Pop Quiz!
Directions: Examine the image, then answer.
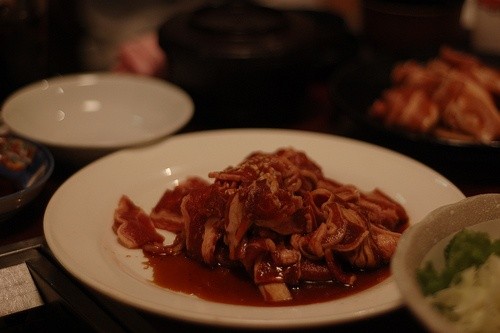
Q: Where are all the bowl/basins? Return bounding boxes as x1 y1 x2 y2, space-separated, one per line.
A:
401 128 500 197
391 191 500 333
1 135 55 221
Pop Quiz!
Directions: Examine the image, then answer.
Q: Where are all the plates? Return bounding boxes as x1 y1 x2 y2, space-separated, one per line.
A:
3 72 195 157
43 128 468 328
